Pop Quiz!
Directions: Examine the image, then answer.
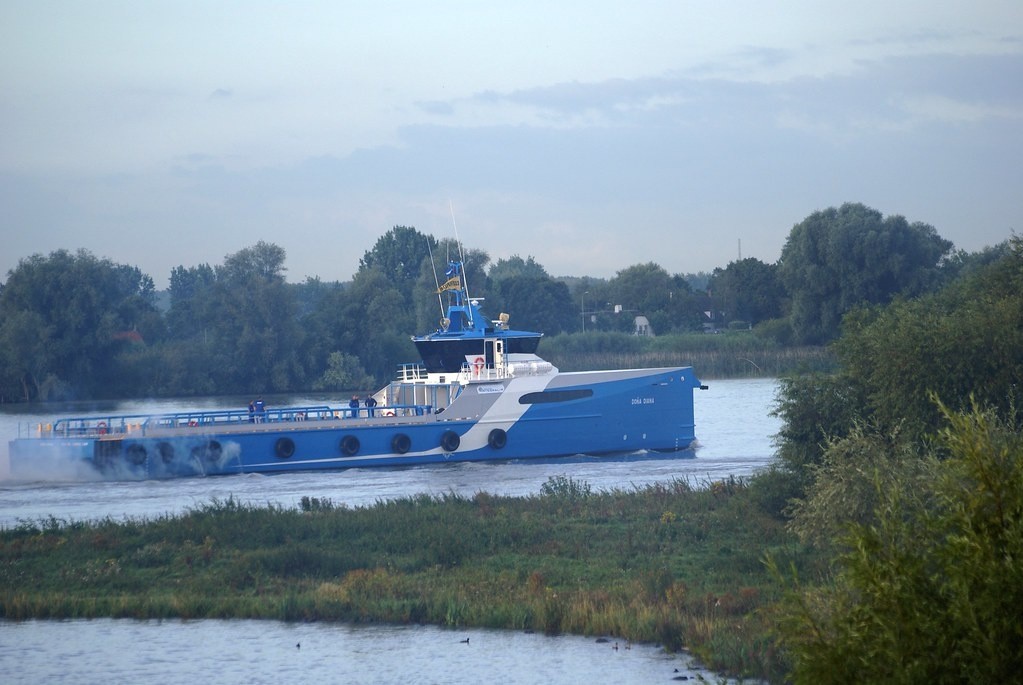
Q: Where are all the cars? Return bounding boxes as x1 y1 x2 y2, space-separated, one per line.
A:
700 327 719 334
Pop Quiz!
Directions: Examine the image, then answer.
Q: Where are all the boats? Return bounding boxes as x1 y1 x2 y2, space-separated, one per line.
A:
10 202 704 489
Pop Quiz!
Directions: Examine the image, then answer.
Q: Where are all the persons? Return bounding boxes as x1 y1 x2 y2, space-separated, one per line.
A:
365 393 377 417
248 396 266 422
349 393 360 417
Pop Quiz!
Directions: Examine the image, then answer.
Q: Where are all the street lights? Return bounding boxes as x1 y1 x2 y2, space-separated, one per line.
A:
582 290 589 332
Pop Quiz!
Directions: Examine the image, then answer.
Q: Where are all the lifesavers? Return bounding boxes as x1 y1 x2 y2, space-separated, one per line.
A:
386 412 394 416
474 357 485 369
154 442 175 463
487 428 507 450
340 434 361 455
440 430 461 452
275 436 295 459
204 441 223 460
125 443 147 465
96 421 107 434
391 433 411 455
188 421 198 427
